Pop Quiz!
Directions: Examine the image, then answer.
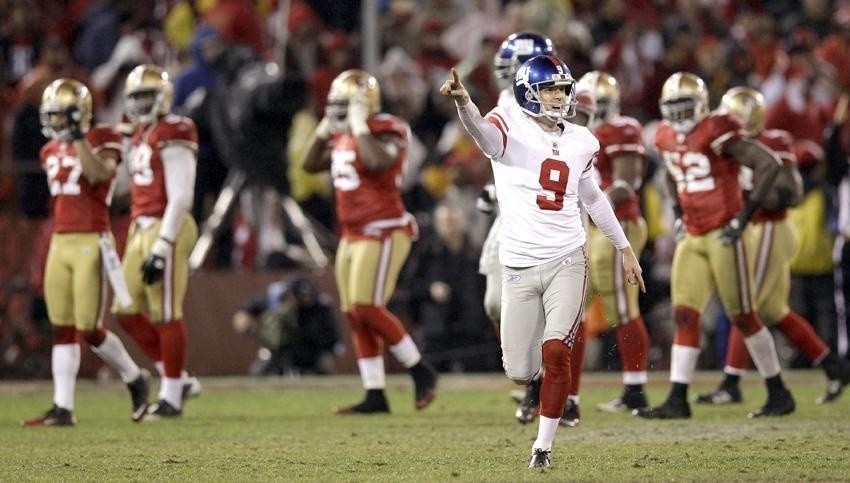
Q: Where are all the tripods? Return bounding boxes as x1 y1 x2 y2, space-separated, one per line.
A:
188 181 338 273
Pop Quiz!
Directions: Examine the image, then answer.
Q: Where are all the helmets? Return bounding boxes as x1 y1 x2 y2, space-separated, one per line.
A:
39 79 93 143
573 70 622 130
494 31 558 80
657 71 710 134
122 64 173 125
324 68 381 136
721 86 768 138
513 55 577 122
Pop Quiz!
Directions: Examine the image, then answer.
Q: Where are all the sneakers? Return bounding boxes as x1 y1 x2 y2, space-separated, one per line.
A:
529 448 552 469
510 373 580 427
632 394 691 419
21 404 78 426
332 388 390 414
815 357 850 405
748 391 794 419
595 390 647 413
696 386 741 406
409 359 438 409
126 369 203 422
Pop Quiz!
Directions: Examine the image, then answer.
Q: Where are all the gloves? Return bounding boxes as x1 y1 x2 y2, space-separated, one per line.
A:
717 214 748 246
139 255 166 285
61 105 86 140
346 95 372 137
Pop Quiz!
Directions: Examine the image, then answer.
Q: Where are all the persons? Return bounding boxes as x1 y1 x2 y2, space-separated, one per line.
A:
0 2 850 467
